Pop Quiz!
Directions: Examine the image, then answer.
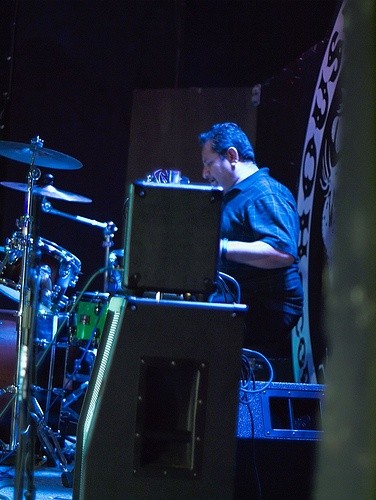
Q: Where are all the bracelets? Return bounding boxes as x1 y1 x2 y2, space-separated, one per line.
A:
221 236 229 261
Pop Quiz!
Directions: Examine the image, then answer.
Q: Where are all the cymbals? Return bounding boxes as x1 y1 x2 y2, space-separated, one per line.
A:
0 139 84 172
0 180 93 204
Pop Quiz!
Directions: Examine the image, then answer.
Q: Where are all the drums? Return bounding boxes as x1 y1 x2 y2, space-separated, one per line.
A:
70 289 110 345
1 311 74 436
1 226 83 316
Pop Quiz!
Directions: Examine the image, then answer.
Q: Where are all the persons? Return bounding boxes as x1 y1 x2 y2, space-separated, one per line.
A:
200 122 303 500
